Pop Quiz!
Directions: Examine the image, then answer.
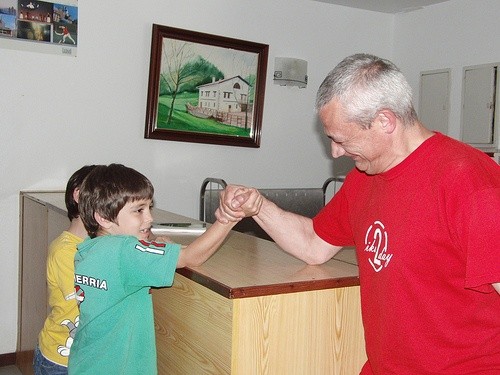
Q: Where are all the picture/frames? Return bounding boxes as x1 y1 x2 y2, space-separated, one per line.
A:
141 21 271 149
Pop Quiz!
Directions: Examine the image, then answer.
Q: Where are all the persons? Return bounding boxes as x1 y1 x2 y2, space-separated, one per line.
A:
215 50 500 375
33 163 103 375
65 162 262 375
0 0 77 46
